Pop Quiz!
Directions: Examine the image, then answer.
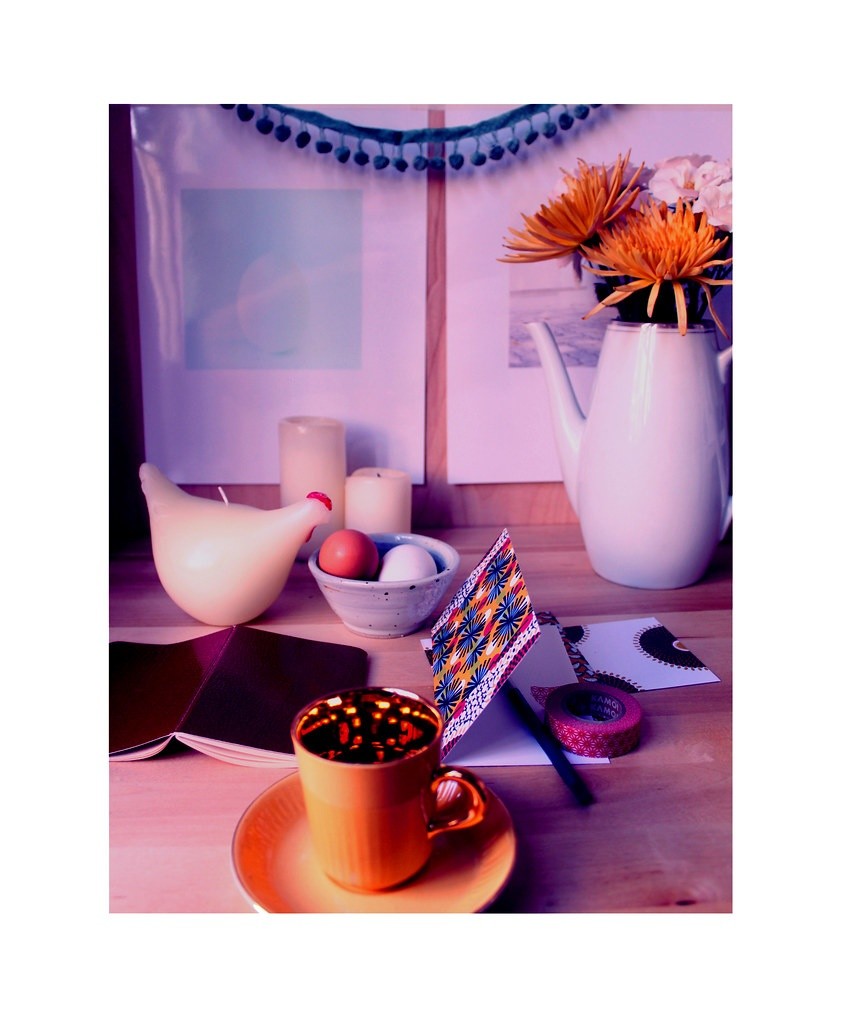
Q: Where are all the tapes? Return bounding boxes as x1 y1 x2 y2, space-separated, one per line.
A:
545 681 645 760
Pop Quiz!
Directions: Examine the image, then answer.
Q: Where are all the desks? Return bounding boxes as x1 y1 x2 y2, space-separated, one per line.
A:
110 521 732 913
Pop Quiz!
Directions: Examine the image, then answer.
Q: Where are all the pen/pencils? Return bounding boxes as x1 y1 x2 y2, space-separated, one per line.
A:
500 681 597 809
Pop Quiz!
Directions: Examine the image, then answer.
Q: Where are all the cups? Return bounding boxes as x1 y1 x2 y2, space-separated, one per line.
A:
292 685 490 894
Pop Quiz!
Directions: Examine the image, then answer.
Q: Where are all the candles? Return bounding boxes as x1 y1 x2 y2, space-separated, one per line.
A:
343 466 412 537
279 414 348 562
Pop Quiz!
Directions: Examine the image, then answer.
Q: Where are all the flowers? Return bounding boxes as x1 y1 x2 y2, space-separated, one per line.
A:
494 149 732 344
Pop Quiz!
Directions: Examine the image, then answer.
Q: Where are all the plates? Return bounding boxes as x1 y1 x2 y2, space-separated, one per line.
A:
231 765 518 913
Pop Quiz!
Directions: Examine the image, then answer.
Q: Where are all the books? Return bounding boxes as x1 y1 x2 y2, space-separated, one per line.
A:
109 623 371 771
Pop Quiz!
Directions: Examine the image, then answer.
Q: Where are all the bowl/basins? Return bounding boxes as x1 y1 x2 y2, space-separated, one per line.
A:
308 531 460 640
